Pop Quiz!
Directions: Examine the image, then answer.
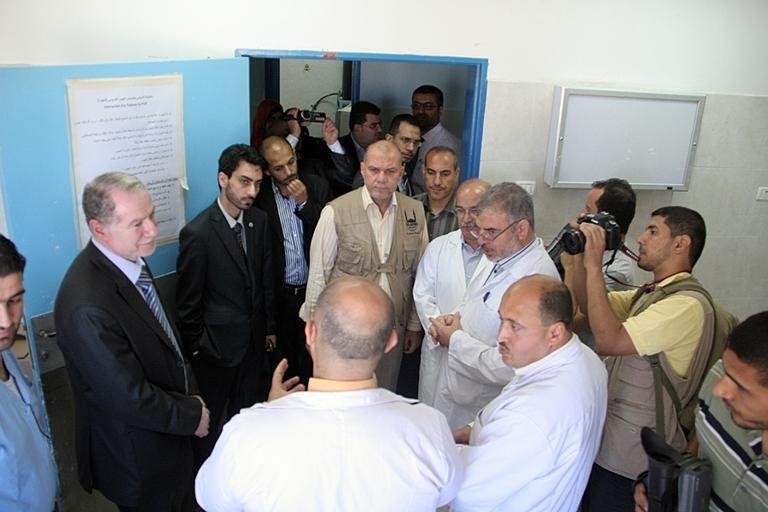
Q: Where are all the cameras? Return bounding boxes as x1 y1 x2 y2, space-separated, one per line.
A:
562 211 622 256
297 109 326 122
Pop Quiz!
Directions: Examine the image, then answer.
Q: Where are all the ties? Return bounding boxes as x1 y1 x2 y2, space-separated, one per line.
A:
229 221 250 264
133 263 185 365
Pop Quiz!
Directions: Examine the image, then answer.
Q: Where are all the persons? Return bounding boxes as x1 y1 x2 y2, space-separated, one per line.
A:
567 207 716 512
52 171 210 511
0 232 63 512
632 309 768 512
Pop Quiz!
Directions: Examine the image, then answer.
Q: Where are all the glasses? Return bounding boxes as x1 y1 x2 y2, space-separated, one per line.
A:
362 120 383 129
410 101 438 112
469 224 503 244
398 135 424 148
